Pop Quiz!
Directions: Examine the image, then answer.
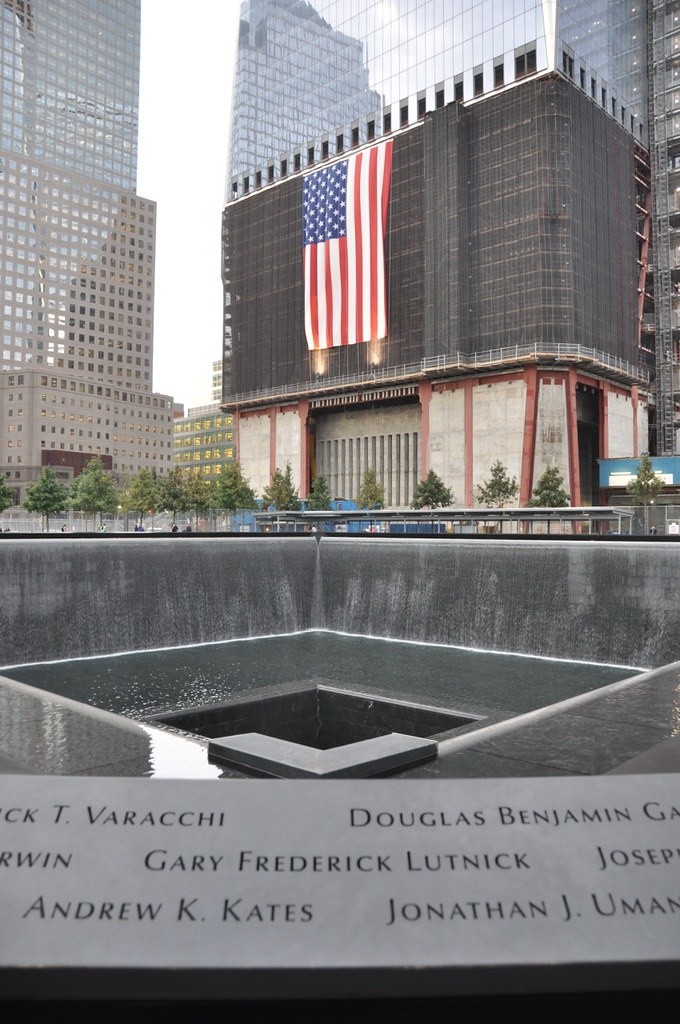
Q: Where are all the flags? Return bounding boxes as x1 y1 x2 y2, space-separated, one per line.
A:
298 138 400 350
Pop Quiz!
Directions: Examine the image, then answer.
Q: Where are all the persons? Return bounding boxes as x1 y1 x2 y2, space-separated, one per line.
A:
649 525 658 537
61 524 66 532
4 525 10 532
309 525 318 533
138 524 144 532
99 524 103 532
605 527 626 537
186 526 192 532
171 524 178 532
279 526 284 531
134 525 138 532
102 523 107 533
